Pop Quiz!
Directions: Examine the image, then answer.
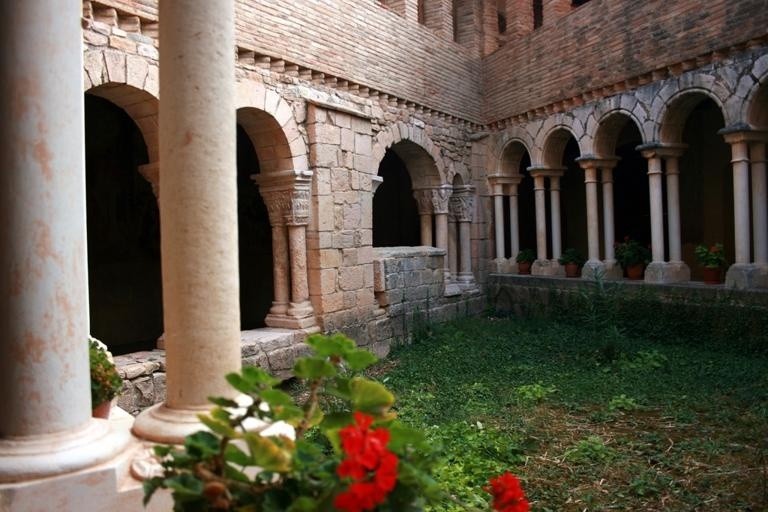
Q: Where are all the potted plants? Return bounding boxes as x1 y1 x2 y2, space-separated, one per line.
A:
614 243 648 281
87 336 126 419
694 243 727 285
515 250 535 273
558 247 583 278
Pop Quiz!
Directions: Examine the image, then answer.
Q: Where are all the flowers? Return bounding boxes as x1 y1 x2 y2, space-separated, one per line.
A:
139 332 534 511
